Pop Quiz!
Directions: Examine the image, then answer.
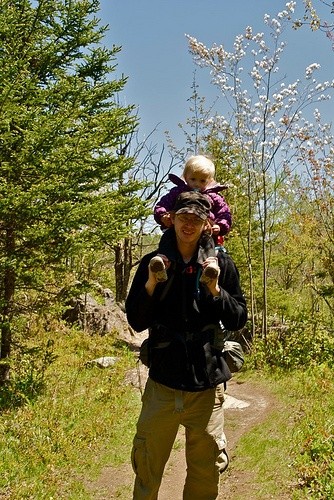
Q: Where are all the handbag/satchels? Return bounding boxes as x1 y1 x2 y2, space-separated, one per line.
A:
222 341 244 373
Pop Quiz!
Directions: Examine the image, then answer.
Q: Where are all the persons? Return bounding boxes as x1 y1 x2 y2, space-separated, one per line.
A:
148 155 232 284
124 190 248 500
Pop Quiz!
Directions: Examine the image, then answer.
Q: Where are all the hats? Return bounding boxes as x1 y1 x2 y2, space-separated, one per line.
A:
174 191 211 221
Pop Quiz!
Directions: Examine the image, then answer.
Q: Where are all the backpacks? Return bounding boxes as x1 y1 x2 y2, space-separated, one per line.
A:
134 338 150 368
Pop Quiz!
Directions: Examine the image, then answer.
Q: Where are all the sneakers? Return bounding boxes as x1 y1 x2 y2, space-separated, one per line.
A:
150 256 167 282
200 256 221 283
